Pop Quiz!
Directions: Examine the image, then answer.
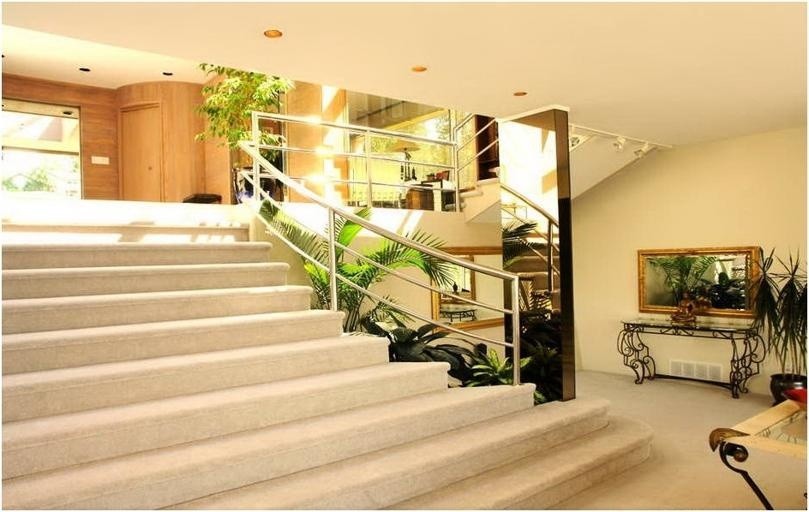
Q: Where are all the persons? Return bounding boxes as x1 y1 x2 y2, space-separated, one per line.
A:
669 290 696 322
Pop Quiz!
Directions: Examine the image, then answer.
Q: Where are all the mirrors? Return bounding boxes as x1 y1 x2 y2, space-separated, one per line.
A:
638 245 760 318
439 257 476 304
431 247 505 335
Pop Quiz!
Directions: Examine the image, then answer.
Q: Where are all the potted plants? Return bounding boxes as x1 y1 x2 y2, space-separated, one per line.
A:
741 246 807 404
647 255 716 322
195 64 294 203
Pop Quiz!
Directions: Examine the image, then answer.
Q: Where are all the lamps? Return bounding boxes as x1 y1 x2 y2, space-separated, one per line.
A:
383 131 421 182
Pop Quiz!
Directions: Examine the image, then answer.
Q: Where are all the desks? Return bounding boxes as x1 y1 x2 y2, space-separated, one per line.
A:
617 318 765 398
440 306 477 322
